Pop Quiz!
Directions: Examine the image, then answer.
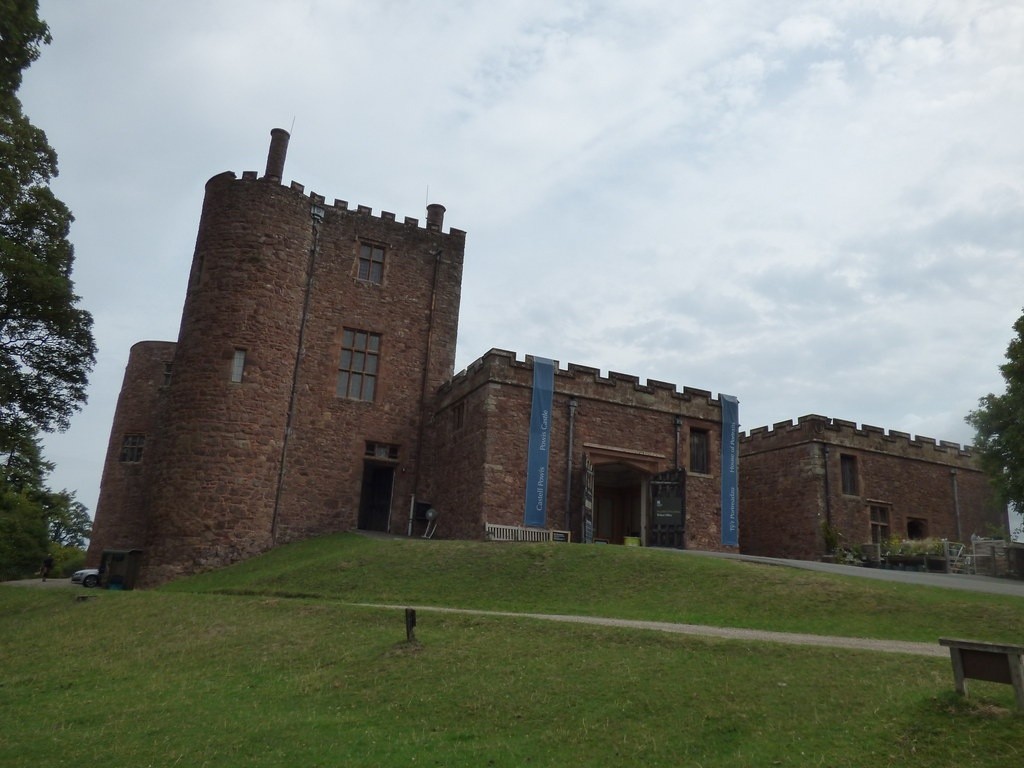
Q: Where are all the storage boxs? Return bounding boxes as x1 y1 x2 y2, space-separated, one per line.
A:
624 536 640 546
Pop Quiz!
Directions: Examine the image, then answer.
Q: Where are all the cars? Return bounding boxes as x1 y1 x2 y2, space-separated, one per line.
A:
71 568 100 589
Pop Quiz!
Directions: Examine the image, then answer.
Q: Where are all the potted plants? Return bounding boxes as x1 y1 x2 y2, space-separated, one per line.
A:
912 540 927 564
885 530 903 569
950 544 959 556
821 521 846 563
901 551 920 571
926 536 947 573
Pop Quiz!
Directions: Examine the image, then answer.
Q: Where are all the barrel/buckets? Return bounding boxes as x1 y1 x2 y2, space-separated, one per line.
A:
624 537 640 546
863 543 880 559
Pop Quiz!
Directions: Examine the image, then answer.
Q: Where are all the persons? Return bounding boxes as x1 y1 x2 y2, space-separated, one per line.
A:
40 553 53 582
